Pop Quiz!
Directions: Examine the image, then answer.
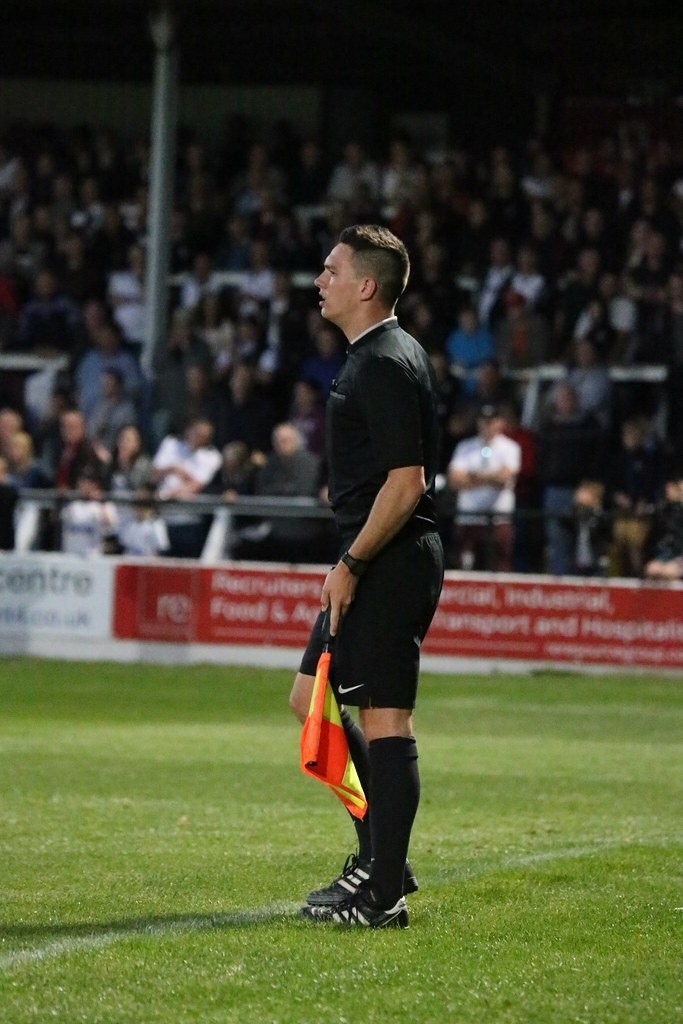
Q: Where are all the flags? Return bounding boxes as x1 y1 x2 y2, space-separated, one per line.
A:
301 652 368 820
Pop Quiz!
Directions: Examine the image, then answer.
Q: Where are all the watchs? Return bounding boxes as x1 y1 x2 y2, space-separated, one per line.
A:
341 552 367 577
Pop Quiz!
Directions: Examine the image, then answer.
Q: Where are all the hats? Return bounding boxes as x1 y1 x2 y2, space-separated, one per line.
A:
478 399 498 421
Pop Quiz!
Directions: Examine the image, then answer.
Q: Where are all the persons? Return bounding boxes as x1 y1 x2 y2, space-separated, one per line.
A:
288 225 443 932
0 94 683 577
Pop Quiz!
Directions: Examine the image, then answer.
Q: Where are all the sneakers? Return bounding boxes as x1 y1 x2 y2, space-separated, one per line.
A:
306 848 418 906
300 886 410 933
